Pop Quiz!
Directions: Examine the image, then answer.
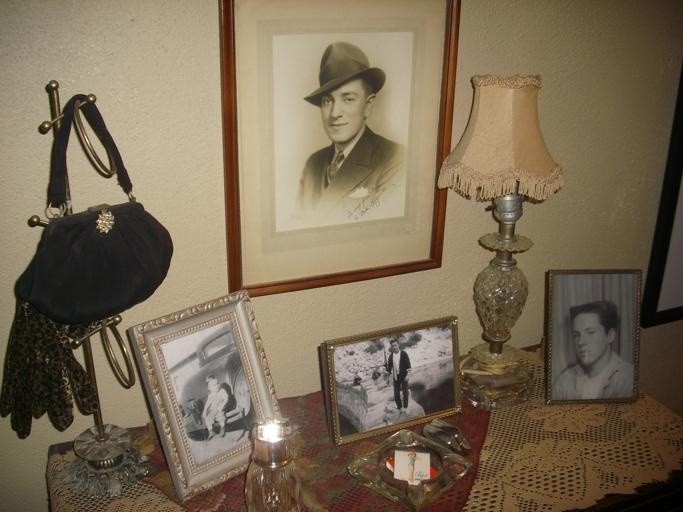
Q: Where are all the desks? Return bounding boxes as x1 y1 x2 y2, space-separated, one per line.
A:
46 344 683 511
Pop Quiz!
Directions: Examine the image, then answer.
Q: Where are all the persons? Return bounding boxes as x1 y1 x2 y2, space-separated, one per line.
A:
551 300 633 398
381 339 412 415
295 39 405 211
201 375 230 440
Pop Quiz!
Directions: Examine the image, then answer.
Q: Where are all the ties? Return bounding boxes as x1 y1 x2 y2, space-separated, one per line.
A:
325 150 344 186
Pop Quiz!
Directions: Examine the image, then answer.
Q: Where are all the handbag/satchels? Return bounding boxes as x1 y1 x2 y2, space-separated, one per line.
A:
14 94 174 324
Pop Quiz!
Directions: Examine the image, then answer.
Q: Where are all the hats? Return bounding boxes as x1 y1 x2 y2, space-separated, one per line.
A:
303 41 386 107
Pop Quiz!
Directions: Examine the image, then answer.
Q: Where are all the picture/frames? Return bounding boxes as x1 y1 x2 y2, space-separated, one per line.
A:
319 316 462 444
546 269 641 403
131 289 283 502
220 1 460 297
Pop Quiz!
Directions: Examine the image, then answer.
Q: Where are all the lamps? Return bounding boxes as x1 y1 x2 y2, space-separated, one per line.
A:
245 418 307 512
437 73 566 412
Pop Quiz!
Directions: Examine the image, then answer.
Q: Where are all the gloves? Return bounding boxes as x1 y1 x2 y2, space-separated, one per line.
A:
0 298 99 440
